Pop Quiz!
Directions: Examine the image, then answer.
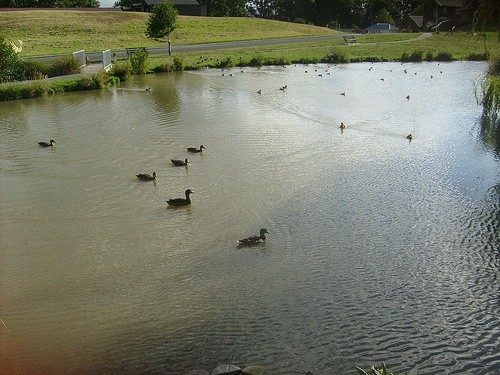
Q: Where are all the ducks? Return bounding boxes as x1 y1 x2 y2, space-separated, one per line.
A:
407 134 412 139
406 95 410 99
340 122 346 128
38 139 56 147
165 189 194 206
257 85 290 96
135 172 157 181
219 67 245 77
186 144 206 153
171 158 188 166
145 88 151 90
237 228 271 244
340 92 346 96
282 63 443 81
